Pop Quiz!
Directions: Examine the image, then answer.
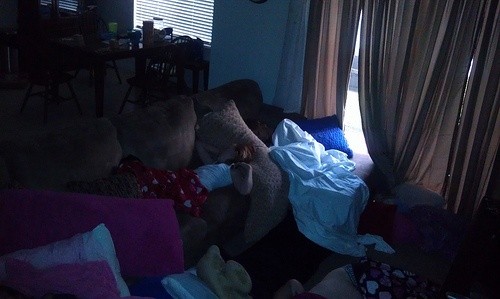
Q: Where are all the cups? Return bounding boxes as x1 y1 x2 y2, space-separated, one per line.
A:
143 21 153 36
108 22 117 33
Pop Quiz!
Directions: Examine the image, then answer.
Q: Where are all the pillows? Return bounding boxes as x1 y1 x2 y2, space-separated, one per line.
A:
294 115 354 158
195 101 286 189
361 184 483 262
0 187 220 299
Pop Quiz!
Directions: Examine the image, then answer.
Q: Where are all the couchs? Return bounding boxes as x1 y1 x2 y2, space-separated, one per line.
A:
0 77 306 268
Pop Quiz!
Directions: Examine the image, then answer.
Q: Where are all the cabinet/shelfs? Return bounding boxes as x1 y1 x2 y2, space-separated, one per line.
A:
16 0 97 74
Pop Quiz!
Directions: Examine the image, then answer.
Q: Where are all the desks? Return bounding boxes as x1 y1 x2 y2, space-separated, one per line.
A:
48 33 184 118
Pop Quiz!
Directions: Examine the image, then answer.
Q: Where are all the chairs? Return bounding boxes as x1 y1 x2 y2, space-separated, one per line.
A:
19 34 83 123
72 11 123 85
117 35 210 115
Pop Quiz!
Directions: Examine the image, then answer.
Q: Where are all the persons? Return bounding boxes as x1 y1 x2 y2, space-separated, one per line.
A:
111 140 258 217
